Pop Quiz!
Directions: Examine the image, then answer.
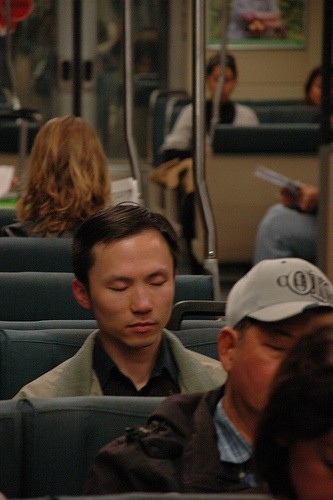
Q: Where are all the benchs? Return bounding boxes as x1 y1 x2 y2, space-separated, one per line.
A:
0 208 230 500
151 89 320 263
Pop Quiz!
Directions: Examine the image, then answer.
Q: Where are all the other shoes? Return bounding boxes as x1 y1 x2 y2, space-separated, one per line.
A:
189 256 210 276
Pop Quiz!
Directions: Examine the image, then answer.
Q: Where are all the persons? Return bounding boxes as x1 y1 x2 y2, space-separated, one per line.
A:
12 199 236 405
0 52 320 277
229 0 285 39
96 258 333 499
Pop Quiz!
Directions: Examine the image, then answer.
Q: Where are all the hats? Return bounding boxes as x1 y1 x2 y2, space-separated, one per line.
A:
225 257 333 329
